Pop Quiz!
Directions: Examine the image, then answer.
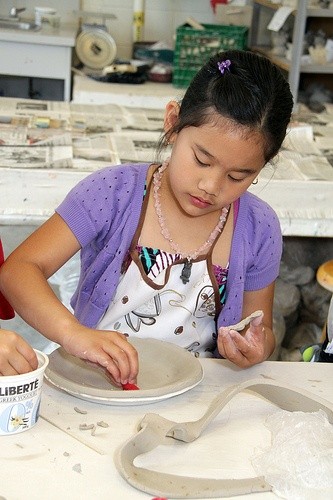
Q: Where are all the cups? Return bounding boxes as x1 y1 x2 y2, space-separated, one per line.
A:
0 347 49 436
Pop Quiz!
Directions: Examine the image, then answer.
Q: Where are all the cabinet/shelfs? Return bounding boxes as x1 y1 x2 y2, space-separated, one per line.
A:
249 0 333 116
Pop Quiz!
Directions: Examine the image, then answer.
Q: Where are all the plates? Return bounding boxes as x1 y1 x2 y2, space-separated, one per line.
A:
144 64 173 83
44 337 204 407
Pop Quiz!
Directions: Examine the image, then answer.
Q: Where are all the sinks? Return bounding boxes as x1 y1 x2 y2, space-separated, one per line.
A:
0 20 40 31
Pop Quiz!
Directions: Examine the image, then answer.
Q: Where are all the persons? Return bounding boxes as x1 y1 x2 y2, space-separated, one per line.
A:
0 238 38 375
0 48 294 385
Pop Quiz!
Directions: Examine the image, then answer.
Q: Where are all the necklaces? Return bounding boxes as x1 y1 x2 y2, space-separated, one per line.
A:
152 157 229 262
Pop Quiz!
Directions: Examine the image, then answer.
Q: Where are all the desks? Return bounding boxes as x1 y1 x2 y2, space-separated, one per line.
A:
0 96 333 352
0 358 333 500
0 18 80 102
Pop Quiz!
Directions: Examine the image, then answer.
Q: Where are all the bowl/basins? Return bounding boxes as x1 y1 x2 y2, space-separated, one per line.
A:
132 41 174 63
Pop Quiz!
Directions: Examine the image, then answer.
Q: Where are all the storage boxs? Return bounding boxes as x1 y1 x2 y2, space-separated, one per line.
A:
172 21 250 89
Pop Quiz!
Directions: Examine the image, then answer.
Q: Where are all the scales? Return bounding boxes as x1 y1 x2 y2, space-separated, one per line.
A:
72 10 118 75
